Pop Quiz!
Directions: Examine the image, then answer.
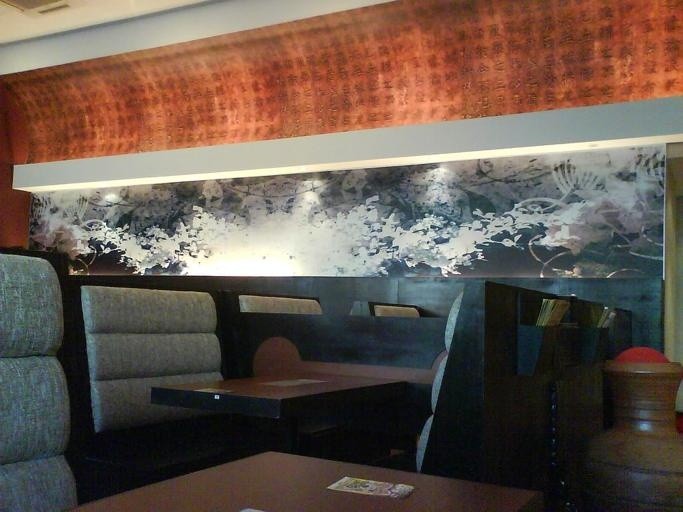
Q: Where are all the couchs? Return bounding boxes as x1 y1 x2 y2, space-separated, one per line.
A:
82 286 635 479
0 254 81 512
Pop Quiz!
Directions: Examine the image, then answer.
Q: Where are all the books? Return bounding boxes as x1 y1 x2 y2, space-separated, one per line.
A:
591 304 617 328
535 298 570 329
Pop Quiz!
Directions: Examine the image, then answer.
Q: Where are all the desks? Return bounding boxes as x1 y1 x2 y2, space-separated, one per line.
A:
72 454 538 512
